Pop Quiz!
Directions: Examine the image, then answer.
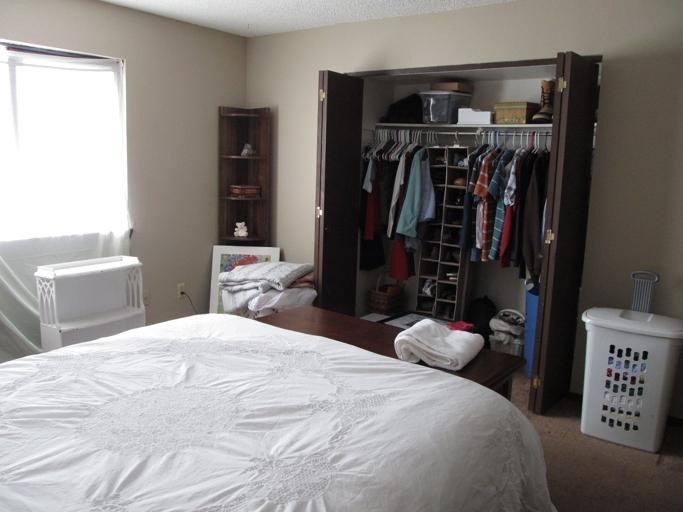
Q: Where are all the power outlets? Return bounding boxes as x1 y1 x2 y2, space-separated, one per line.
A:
176 282 186 299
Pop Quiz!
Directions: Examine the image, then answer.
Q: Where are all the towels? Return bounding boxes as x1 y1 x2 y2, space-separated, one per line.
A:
393 317 486 374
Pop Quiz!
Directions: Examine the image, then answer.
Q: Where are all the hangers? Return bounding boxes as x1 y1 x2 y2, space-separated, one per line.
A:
360 128 549 163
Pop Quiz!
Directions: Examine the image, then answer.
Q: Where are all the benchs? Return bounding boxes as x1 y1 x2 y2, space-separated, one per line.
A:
254 303 527 403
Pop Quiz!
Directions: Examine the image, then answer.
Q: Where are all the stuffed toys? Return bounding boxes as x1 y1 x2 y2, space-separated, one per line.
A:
233 220 247 238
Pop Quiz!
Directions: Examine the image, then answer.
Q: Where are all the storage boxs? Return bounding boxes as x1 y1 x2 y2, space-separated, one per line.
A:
415 81 540 124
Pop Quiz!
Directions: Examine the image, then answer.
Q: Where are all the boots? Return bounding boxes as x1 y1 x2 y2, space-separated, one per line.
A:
532 80 553 123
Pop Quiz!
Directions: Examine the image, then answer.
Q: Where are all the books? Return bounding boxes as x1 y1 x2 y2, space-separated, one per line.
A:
358 311 390 323
229 184 259 199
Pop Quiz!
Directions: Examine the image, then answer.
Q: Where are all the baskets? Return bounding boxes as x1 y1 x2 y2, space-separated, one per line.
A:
489 334 523 357
366 268 406 316
580 306 683 454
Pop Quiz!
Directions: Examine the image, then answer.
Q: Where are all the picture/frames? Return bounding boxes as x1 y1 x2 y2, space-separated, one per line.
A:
208 244 281 314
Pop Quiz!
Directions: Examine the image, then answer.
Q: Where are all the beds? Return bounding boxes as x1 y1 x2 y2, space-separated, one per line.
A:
0 311 559 512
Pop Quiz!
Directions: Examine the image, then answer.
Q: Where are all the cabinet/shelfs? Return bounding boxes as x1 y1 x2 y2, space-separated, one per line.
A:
415 145 478 322
32 254 147 351
217 103 273 247
